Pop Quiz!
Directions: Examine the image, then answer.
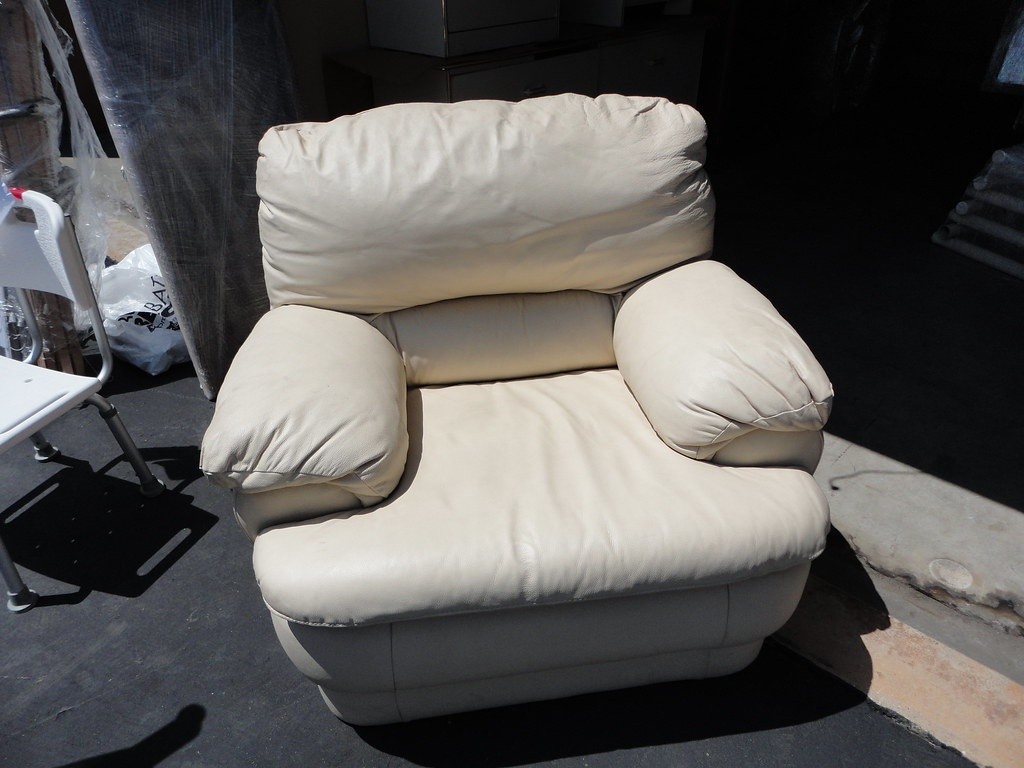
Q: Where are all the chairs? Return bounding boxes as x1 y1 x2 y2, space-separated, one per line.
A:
0 187 168 614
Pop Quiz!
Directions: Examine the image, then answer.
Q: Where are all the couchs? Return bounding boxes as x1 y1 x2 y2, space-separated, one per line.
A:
198 94 838 725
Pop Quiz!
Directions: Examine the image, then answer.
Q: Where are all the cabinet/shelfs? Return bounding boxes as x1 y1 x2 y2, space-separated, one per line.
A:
371 1 709 109
